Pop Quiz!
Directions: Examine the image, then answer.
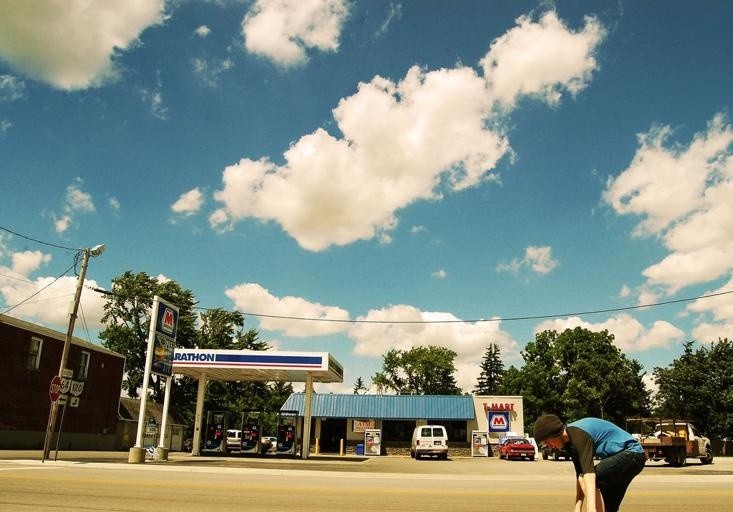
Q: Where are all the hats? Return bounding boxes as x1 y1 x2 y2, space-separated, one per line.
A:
532 415 565 445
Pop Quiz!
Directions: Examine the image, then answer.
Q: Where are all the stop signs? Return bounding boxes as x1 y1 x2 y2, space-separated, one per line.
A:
47 376 61 400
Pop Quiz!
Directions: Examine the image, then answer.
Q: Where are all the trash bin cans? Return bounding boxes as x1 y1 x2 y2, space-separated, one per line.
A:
356 443 364 455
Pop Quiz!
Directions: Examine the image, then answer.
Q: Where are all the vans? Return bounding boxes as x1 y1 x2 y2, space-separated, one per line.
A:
410 425 449 460
225 430 241 448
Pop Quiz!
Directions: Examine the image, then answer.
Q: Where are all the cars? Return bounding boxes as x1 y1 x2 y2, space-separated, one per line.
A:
499 438 535 461
542 445 571 461
259 436 277 451
183 437 191 451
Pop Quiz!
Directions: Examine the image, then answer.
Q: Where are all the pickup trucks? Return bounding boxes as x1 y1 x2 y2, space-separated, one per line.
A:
624 418 714 468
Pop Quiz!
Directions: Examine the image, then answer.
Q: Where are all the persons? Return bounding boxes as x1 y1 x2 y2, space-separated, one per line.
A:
534 414 646 512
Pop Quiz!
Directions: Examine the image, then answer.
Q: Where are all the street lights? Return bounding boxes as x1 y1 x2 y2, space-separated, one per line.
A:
39 242 105 460
